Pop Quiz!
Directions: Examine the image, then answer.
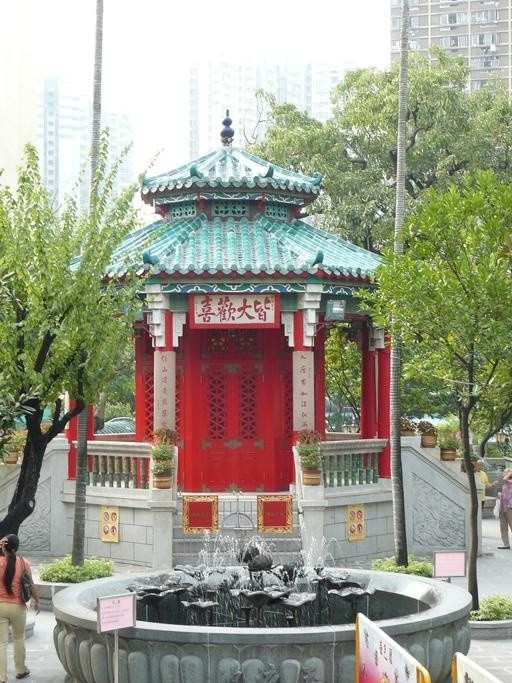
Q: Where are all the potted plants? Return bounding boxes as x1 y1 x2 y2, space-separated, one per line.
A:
149 428 177 488
298 429 322 485
400 416 479 472
5 438 22 463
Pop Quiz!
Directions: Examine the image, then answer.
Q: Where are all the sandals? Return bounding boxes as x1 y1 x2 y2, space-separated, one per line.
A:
16 672 30 679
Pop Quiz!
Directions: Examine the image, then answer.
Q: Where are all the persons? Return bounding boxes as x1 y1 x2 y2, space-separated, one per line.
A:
497 467 512 549
474 459 499 510
0 534 41 683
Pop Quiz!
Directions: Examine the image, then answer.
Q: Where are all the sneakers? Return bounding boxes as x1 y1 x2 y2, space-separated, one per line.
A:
498 546 510 549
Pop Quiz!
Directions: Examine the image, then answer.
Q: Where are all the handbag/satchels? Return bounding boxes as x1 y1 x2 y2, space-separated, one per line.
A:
20 556 31 602
493 499 500 518
